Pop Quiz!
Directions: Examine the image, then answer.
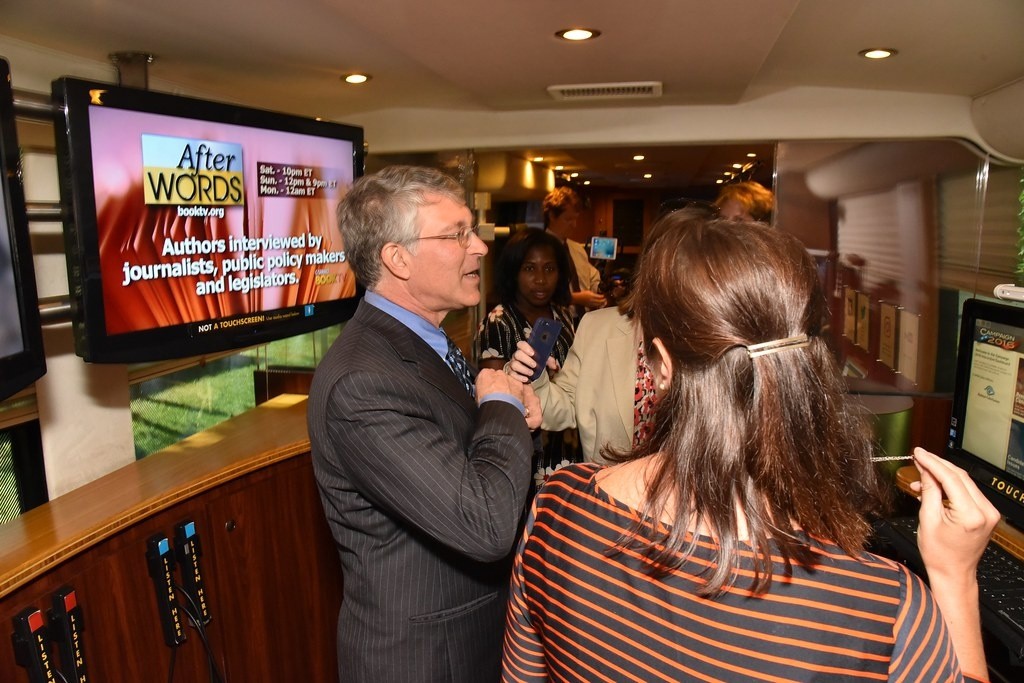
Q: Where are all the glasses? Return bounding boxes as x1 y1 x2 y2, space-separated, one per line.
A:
418 222 479 249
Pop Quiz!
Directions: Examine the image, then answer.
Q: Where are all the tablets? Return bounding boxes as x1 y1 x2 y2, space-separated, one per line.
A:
590 236 617 260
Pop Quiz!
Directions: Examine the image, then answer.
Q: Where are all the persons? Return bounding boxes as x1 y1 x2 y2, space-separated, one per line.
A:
717 180 781 228
472 226 587 523
542 188 609 334
305 167 543 683
499 209 1001 683
508 204 722 474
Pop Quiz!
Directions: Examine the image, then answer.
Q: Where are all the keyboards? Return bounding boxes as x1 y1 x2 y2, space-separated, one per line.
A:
861 513 1024 659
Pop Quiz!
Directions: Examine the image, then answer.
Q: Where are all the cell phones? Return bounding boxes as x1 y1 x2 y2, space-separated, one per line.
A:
517 317 562 383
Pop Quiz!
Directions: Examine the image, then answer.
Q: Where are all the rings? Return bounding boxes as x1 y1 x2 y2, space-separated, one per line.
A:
525 407 531 419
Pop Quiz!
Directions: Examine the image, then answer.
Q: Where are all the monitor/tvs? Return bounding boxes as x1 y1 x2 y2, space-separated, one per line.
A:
0 55 48 402
947 298 1024 532
51 75 364 363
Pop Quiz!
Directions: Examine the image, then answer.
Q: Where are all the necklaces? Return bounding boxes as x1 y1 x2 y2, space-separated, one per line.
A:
848 456 917 463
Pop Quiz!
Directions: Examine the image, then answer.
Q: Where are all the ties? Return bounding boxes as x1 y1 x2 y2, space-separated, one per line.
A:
442 332 475 401
